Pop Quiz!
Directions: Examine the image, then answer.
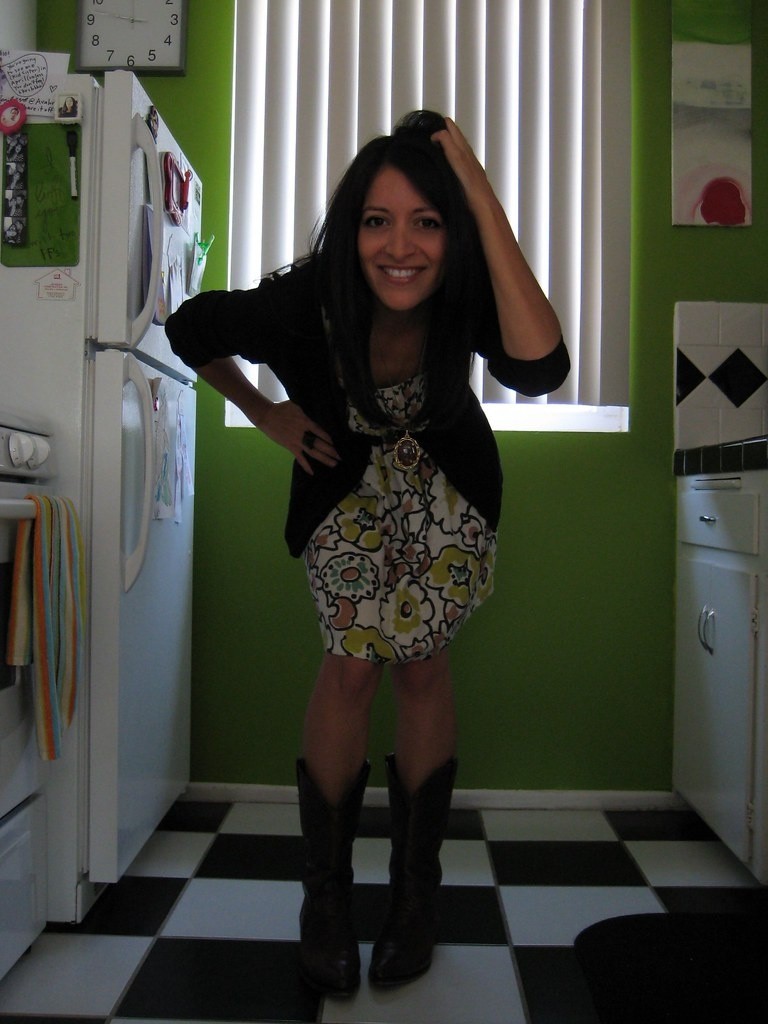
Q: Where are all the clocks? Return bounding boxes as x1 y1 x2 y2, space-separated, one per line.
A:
75 0 188 76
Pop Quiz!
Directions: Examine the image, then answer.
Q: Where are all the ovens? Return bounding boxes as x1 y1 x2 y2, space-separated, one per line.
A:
0 482 77 839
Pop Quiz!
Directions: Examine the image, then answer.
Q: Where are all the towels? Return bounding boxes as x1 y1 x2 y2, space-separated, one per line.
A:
5 493 89 762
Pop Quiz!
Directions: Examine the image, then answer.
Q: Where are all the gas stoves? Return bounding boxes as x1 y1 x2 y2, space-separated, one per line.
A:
0 411 59 479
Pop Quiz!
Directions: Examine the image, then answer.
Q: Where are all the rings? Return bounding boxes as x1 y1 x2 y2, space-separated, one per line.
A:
302 430 316 450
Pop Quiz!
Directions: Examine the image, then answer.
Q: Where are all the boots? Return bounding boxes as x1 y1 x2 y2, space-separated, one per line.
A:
367 753 458 990
296 756 370 999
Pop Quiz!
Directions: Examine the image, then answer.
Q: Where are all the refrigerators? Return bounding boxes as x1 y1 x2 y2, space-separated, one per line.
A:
0 70 205 922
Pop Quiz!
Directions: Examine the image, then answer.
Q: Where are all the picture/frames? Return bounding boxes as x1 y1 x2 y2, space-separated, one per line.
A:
0 99 27 136
54 92 82 121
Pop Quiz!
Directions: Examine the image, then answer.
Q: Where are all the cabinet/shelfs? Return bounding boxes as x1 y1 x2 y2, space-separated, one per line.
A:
674 469 760 882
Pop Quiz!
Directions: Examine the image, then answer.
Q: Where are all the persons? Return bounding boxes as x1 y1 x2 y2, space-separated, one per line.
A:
164 107 570 1001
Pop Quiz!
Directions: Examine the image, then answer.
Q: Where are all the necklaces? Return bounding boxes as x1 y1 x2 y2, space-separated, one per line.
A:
394 430 421 469
